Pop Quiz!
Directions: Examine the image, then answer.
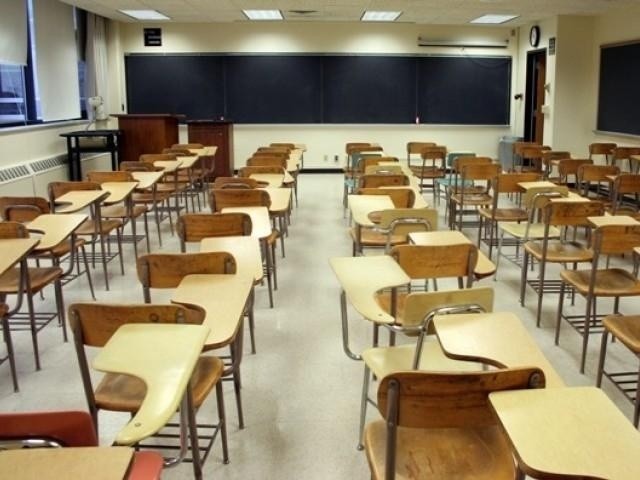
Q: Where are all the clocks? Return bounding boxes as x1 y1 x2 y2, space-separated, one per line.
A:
528 24 540 47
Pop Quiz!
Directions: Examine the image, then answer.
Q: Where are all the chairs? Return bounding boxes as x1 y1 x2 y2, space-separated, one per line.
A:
120 143 213 246
208 189 285 291
406 142 436 172
0 304 20 393
68 302 230 480
357 287 493 451
373 244 478 347
493 185 568 281
595 314 640 429
0 197 96 327
444 156 492 226
450 164 503 240
604 173 640 218
610 147 640 174
0 221 66 371
0 411 164 480
351 209 438 257
214 143 308 208
519 146 552 207
434 153 477 207
554 224 640 375
47 181 125 292
577 165 620 201
342 143 415 226
520 201 605 328
588 143 617 166
538 150 571 182
477 173 543 260
87 171 150 259
418 145 446 194
360 366 545 479
176 212 274 309
558 159 594 189
136 251 257 429
507 142 541 201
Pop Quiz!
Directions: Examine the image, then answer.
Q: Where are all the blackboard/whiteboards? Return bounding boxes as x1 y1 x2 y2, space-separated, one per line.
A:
121 52 514 128
588 40 640 146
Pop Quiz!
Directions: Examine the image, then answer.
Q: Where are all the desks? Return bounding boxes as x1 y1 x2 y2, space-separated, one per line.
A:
61 129 122 182
